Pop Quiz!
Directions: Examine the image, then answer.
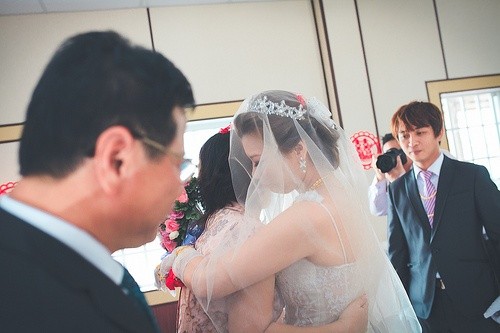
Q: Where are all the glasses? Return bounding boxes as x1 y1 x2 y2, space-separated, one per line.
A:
132 129 199 183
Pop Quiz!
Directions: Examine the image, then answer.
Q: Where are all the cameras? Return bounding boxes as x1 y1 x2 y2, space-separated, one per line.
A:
376 148 407 173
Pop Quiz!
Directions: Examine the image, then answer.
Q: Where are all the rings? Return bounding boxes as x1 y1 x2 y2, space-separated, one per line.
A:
156 263 169 281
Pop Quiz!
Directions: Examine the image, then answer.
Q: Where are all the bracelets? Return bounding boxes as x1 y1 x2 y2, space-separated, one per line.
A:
175 245 195 257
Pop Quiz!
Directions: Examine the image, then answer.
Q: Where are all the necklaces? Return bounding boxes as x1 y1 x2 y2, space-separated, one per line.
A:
427 213 435 218
302 175 325 193
419 191 437 200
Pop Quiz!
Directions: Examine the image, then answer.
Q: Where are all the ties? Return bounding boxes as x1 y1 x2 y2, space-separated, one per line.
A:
419 170 438 230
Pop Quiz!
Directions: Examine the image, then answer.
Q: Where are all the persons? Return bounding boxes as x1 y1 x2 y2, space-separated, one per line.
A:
369 132 413 216
175 131 368 333
154 90 423 333
0 30 196 333
386 100 500 333
